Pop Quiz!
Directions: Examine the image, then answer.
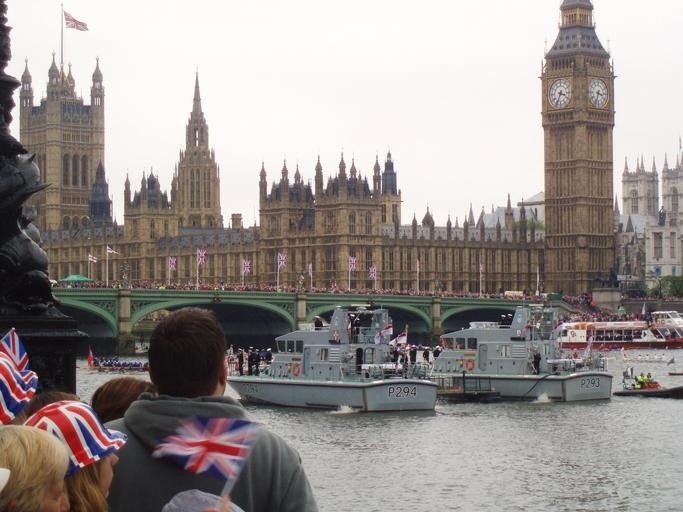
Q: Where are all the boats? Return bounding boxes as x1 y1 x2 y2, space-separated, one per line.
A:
88 366 149 372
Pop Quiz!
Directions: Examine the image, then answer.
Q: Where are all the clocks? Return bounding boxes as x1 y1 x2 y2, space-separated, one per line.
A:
547 78 572 109
588 77 610 108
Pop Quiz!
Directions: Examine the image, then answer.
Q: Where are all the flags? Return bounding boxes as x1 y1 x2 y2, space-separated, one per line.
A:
0 330 38 424
89 254 98 263
169 257 177 271
198 249 206 265
278 252 287 268
368 264 377 280
349 256 357 272
244 260 251 273
107 245 119 254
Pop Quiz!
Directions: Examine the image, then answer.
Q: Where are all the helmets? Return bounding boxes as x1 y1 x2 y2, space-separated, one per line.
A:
24 400 127 477
0 351 38 428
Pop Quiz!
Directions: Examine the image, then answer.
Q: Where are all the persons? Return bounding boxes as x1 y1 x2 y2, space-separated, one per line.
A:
390 343 443 364
633 372 652 389
532 348 541 375
1 391 118 512
50 281 672 341
234 346 273 376
91 303 319 512
93 355 149 368
